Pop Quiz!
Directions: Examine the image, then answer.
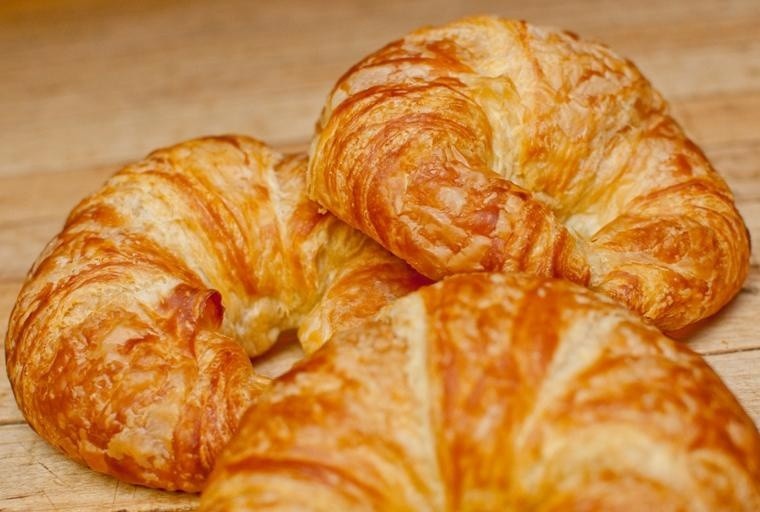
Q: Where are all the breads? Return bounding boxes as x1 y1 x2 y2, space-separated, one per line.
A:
199 272 760 512
5 135 436 492
307 16 751 332
